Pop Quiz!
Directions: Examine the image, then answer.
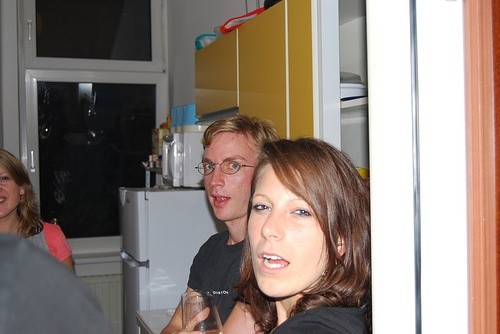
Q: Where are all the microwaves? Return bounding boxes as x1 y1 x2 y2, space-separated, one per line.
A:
162 133 204 189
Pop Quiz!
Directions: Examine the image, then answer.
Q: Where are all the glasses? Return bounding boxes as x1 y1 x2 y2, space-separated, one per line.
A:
194 160 256 175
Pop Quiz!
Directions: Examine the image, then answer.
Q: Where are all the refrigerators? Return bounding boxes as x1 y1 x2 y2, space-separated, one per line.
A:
118 186 227 334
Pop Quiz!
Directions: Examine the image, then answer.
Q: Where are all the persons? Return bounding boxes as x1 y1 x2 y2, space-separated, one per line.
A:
39 103 71 223
160 113 280 334
0 149 74 272
222 137 371 334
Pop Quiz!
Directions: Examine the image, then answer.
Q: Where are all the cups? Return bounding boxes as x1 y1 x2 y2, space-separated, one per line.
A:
181 291 224 334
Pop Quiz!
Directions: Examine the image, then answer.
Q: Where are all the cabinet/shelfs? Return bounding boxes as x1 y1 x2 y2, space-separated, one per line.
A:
195 0 313 140
